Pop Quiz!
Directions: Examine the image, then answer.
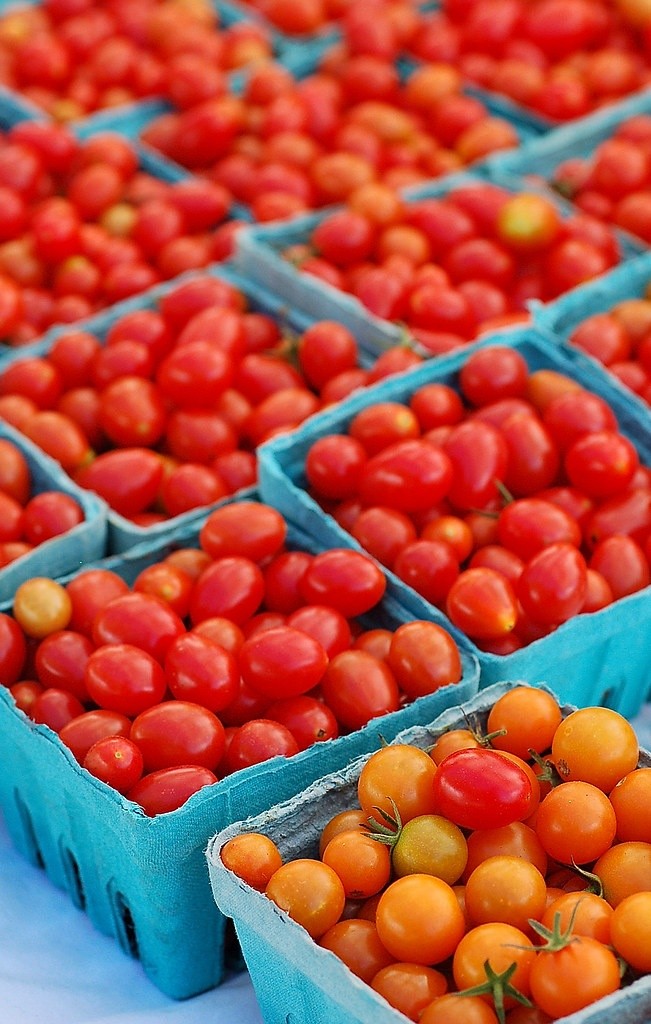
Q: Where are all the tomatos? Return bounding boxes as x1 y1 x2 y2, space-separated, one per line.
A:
0 0 651 1024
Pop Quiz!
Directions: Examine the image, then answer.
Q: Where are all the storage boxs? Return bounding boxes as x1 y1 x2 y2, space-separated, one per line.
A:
0 0 650 1022
